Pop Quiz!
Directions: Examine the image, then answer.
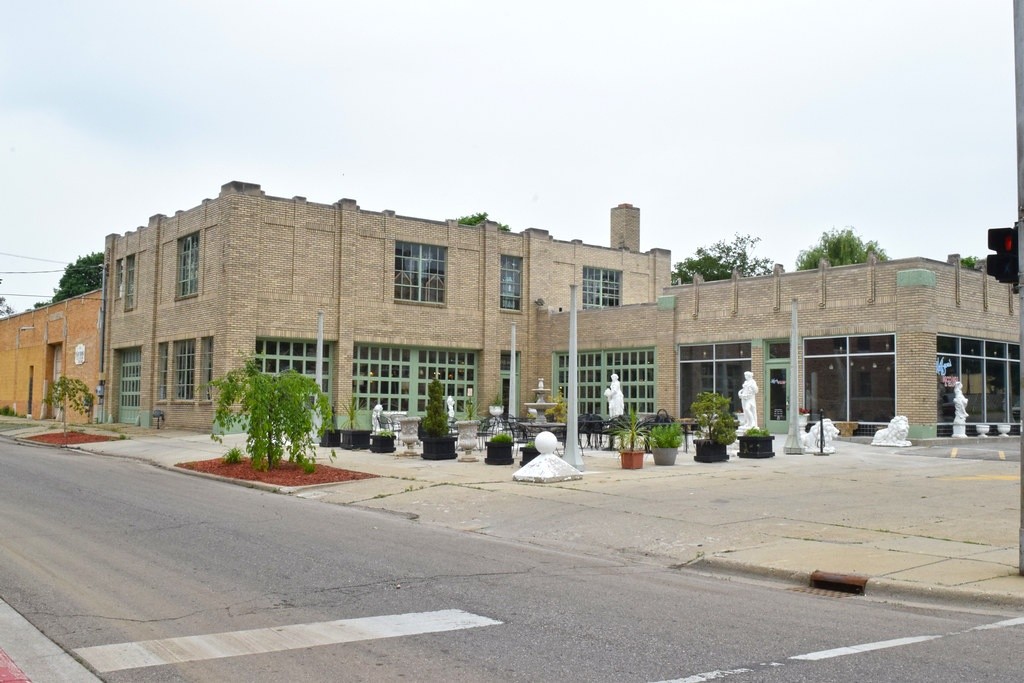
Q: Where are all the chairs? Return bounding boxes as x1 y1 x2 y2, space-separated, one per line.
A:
577 409 675 453
377 413 557 457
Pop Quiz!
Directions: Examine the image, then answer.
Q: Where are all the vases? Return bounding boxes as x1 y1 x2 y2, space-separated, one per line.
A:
397 417 421 438
976 425 990 437
997 424 1011 437
320 429 342 443
799 413 810 427
736 413 746 426
391 411 408 425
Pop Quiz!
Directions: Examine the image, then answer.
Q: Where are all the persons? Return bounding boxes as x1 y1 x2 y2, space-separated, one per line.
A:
604 374 624 418
953 382 969 418
538 376 544 389
737 371 760 430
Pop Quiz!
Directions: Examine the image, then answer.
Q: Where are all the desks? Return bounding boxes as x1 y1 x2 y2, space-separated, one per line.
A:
586 420 620 451
519 422 566 455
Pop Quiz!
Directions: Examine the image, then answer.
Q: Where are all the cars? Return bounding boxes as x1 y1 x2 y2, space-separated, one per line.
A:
562 414 676 435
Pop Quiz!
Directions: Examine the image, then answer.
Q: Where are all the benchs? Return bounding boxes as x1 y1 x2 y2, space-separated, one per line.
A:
835 422 858 437
677 418 694 432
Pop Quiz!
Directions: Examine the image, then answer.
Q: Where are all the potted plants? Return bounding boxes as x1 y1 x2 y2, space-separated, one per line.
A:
604 406 666 469
339 395 373 444
520 441 541 461
690 391 740 457
421 378 458 454
488 393 507 416
525 413 536 421
485 433 515 458
648 423 684 466
454 397 484 449
737 426 774 452
370 430 397 448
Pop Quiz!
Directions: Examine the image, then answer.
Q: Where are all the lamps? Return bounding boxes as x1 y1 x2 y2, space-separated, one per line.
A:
535 298 545 306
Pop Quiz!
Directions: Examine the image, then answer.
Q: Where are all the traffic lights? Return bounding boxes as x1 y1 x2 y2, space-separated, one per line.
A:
985 228 1018 283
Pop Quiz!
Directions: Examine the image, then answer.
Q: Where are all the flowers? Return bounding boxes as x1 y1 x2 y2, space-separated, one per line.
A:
799 408 810 415
737 407 743 413
